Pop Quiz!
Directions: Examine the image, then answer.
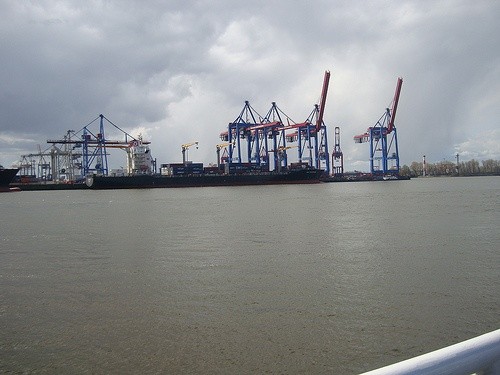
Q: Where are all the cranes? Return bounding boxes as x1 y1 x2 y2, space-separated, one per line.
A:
1 69 403 191
